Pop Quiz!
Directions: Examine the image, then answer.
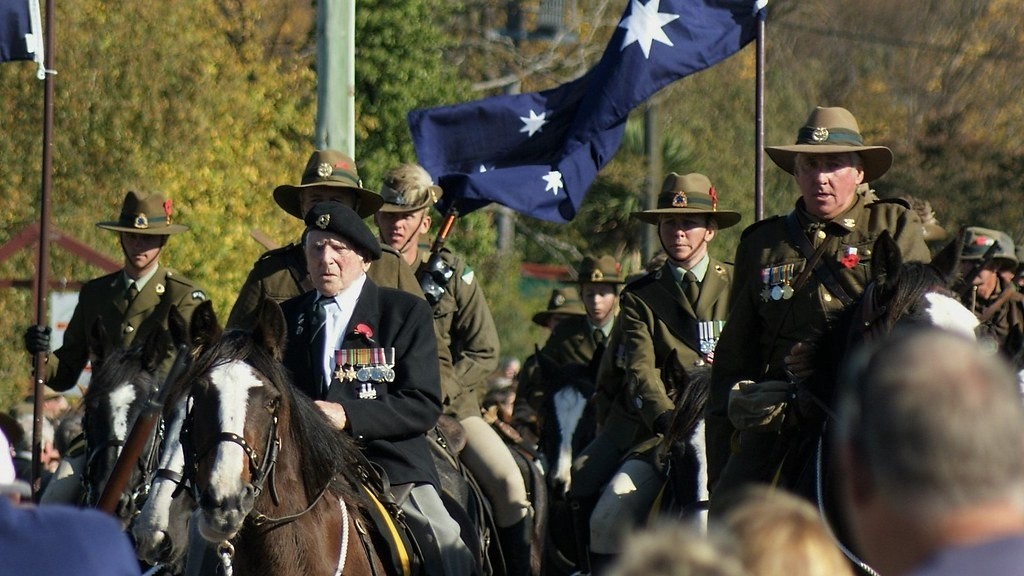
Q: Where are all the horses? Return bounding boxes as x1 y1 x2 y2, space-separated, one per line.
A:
35 230 1024 576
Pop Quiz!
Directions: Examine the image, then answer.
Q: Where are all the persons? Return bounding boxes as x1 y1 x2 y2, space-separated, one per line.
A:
0 107 1024 576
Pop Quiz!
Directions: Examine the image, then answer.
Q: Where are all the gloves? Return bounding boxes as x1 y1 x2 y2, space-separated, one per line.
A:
25 326 52 354
654 411 686 460
727 378 790 433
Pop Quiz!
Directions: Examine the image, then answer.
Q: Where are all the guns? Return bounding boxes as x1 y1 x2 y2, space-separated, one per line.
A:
956 240 1002 297
97 344 189 514
430 200 459 256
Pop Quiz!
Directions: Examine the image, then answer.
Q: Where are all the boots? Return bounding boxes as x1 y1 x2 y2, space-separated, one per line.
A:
493 507 533 576
587 546 622 576
566 490 599 574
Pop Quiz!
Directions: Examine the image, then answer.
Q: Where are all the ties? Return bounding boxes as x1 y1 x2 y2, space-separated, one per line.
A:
128 283 138 306
309 296 334 399
684 271 700 313
594 329 603 346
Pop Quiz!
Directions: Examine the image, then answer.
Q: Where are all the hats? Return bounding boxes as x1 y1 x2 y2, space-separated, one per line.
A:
624 271 649 286
24 384 64 401
306 204 381 260
275 151 384 219
961 227 1018 272
532 287 588 327
764 107 893 184
377 164 444 212
560 255 627 284
630 173 742 230
96 191 189 235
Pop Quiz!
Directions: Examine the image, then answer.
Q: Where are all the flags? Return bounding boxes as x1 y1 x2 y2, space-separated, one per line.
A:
409 0 768 224
